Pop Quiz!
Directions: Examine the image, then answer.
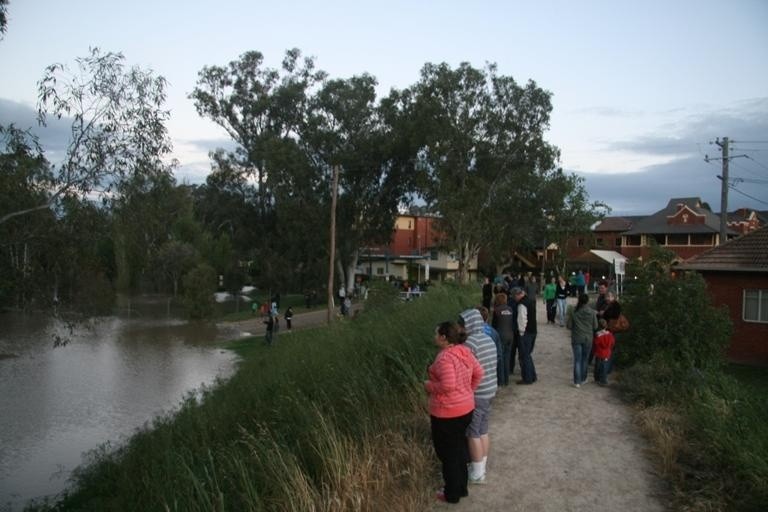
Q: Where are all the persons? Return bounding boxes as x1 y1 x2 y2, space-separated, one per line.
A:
474 269 622 412
458 309 498 485
421 320 483 504
251 276 434 347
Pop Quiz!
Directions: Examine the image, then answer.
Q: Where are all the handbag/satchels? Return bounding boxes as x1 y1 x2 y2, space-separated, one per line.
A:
607 312 630 333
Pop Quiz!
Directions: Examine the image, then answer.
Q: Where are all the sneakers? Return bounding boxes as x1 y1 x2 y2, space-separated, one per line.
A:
436 491 446 500
467 460 475 471
467 471 486 484
